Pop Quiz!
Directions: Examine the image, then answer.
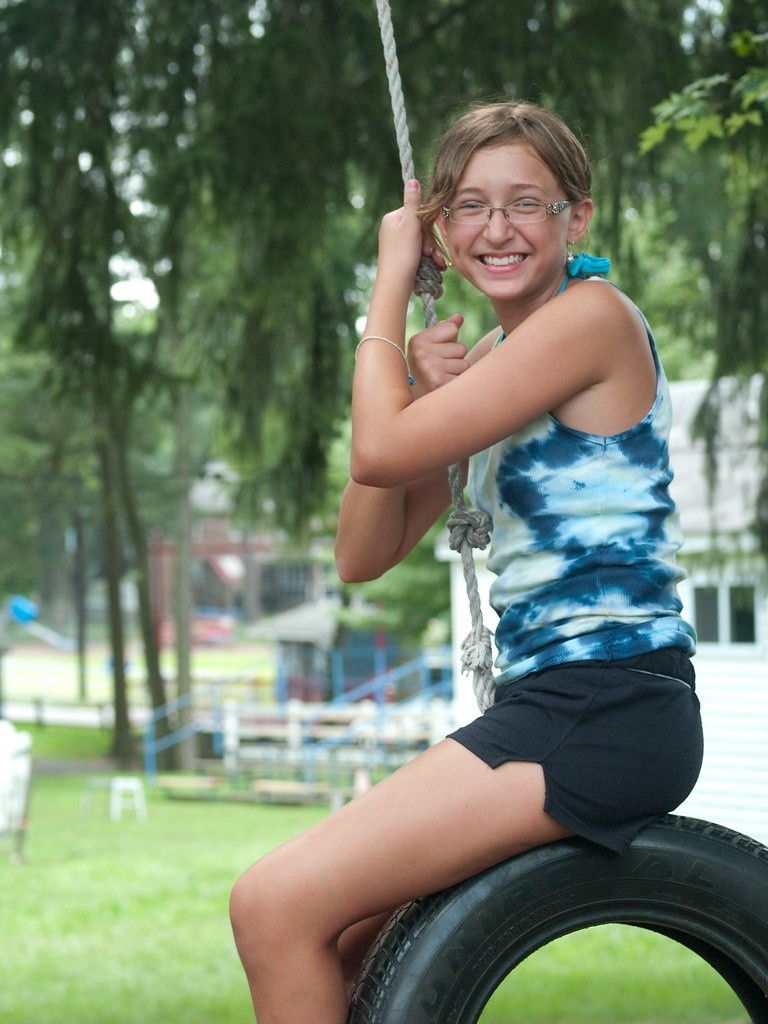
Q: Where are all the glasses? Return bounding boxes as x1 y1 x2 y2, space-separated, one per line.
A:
440 199 575 224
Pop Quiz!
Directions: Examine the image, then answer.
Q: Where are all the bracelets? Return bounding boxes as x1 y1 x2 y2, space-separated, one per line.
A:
355 336 415 385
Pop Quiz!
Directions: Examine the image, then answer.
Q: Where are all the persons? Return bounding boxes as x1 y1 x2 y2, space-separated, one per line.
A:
228 99 704 1024
